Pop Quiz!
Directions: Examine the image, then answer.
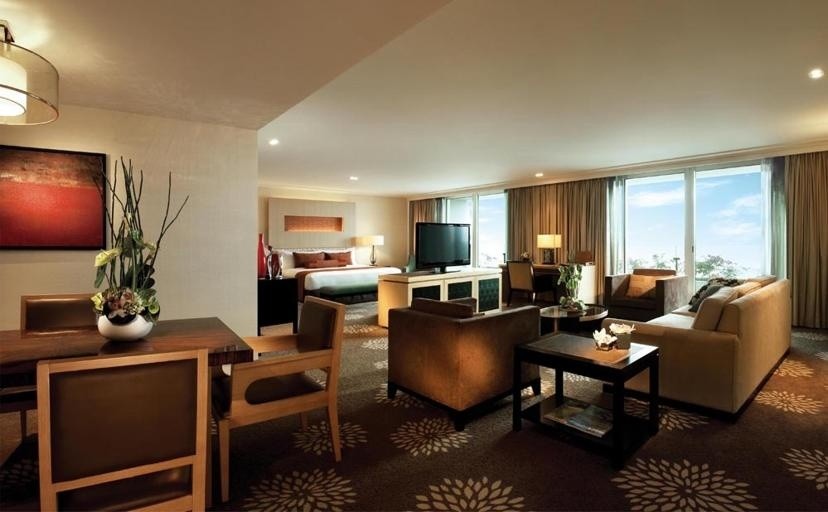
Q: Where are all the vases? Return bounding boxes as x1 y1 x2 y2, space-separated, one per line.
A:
596 344 610 352
613 334 631 350
96 314 152 342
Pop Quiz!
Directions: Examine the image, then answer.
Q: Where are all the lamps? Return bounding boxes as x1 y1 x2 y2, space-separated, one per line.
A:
369 235 384 266
538 234 562 265
0 19 60 125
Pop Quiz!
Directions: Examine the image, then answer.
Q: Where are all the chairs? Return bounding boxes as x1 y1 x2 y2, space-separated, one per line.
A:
388 296 540 430
1 347 209 512
212 296 346 501
603 268 690 321
21 294 96 331
507 261 556 306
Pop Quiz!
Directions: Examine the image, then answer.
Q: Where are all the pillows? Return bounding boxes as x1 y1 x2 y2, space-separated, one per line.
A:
687 274 777 329
625 274 675 297
412 298 474 318
293 251 352 268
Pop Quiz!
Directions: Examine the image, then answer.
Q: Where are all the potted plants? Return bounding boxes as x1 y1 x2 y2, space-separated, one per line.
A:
558 261 588 317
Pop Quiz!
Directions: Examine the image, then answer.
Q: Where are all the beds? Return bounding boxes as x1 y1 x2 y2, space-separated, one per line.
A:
271 247 402 305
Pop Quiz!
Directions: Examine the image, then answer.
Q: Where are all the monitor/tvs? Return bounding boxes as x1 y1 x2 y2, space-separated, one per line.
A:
415 221 471 275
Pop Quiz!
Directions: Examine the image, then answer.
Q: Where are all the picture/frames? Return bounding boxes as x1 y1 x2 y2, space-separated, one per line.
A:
0 143 107 251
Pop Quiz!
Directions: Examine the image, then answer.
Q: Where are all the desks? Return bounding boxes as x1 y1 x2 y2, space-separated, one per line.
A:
513 329 660 472
0 315 253 502
498 264 568 309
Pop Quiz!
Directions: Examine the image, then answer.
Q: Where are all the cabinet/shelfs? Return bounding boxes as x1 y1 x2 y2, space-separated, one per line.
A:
378 267 502 329
258 278 298 336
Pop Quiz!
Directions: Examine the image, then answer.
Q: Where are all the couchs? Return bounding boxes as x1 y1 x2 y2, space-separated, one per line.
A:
600 275 794 414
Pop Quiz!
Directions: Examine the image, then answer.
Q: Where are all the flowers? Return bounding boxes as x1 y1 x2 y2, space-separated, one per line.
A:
591 328 617 344
90 155 190 325
609 322 636 334
